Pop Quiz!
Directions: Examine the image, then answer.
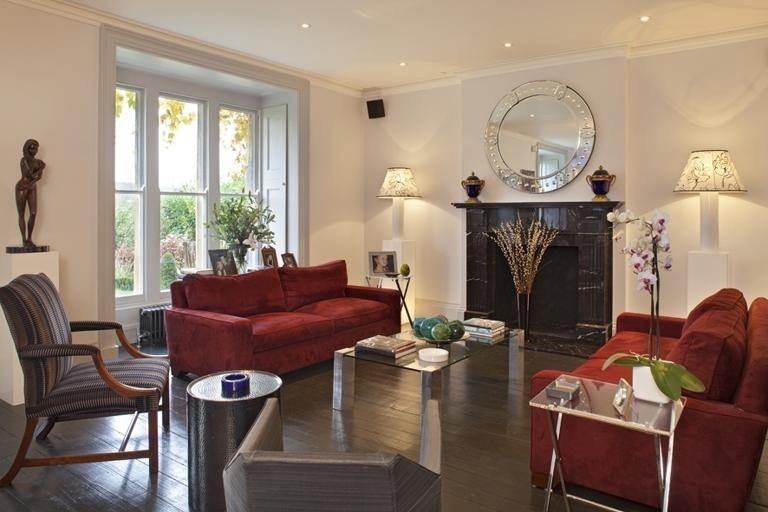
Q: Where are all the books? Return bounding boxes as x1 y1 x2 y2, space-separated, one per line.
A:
354 345 417 358
355 334 417 359
463 317 505 330
463 325 505 334
468 327 505 338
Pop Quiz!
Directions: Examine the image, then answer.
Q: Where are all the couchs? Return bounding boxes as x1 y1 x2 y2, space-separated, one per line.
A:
530 297 768 507
164 282 400 378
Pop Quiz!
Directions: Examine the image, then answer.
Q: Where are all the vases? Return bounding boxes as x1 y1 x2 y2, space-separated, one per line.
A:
517 295 531 338
632 365 671 403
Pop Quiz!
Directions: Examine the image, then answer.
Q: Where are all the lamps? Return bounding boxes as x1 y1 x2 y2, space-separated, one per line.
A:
673 151 747 250
378 166 423 239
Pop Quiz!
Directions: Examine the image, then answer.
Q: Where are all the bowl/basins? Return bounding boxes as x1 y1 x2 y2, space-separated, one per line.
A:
411 328 469 347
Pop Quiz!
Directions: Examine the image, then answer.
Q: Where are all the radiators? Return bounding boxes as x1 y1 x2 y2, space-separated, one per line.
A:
142 306 172 340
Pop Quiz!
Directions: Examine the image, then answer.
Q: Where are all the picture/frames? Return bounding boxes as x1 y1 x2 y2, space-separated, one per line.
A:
209 247 297 274
370 252 398 276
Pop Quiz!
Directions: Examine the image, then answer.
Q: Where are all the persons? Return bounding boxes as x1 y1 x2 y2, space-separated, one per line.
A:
266 255 275 267
374 253 392 272
14 138 46 248
215 255 229 275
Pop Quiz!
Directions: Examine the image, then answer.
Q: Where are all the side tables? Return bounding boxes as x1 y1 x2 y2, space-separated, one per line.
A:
528 375 688 511
368 274 413 328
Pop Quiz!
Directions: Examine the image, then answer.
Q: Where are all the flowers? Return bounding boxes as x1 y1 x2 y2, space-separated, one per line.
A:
606 209 706 399
205 189 276 263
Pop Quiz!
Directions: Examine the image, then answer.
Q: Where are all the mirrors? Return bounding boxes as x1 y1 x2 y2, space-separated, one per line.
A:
484 80 596 193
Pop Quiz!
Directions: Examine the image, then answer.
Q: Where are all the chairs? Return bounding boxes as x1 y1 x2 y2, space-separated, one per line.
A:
223 399 443 512
0 272 169 487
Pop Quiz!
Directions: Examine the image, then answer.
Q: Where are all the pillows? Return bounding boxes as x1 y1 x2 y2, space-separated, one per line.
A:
184 266 287 316
281 259 350 311
666 310 747 402
681 289 747 337
